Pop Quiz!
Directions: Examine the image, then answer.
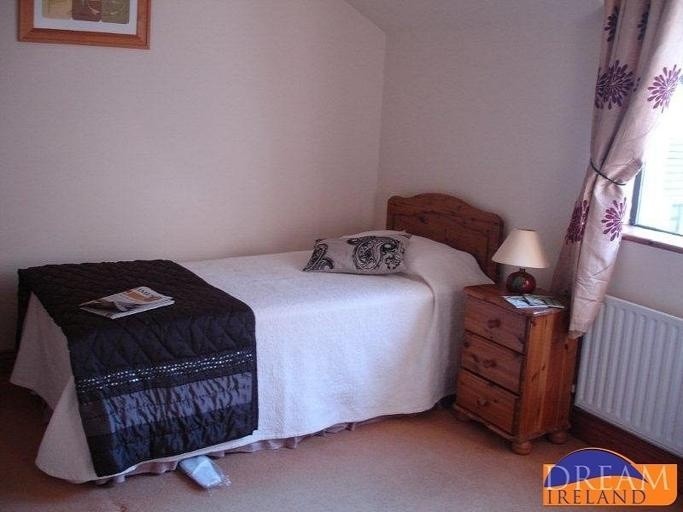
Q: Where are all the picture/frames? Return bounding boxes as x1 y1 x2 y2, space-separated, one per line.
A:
18 0 151 49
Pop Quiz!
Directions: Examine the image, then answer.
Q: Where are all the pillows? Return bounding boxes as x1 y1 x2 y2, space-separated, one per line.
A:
303 231 413 275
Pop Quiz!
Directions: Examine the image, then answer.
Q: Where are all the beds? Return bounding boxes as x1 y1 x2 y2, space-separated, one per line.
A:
8 192 503 485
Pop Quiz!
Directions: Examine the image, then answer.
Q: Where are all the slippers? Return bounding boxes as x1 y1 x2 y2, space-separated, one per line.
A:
178 455 222 490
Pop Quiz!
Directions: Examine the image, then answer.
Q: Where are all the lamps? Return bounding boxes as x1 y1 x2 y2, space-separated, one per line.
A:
492 227 549 295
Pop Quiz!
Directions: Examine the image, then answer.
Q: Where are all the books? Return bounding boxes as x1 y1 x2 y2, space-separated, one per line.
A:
522 292 564 309
497 294 549 310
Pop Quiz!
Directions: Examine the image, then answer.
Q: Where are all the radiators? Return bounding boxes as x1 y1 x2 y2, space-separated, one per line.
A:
573 295 683 457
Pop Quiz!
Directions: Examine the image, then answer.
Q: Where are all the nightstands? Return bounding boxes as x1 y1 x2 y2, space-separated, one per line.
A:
452 283 579 455
